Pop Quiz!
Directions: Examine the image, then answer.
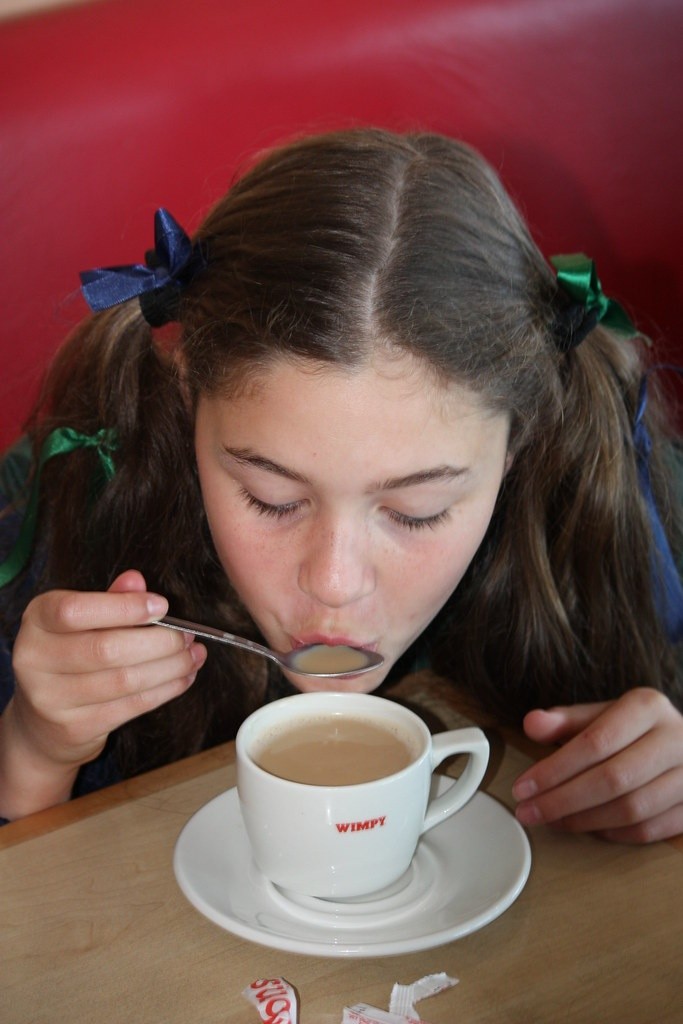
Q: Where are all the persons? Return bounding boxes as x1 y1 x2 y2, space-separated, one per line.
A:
0 129 683 845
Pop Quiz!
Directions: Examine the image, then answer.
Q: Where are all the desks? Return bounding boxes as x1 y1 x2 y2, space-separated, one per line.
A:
0 650 683 1023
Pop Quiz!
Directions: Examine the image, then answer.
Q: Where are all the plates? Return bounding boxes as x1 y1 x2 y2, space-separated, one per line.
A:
172 773 532 959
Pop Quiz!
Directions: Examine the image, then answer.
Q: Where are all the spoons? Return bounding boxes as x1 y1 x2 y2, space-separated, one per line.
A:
152 616 384 677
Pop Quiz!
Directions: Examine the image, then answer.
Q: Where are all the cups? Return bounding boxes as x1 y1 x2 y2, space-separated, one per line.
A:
235 691 491 899
